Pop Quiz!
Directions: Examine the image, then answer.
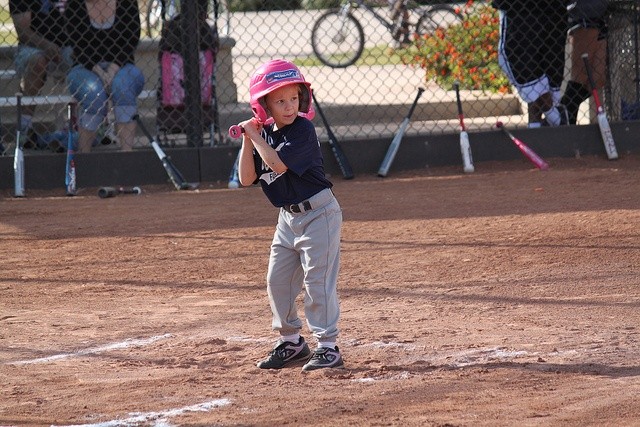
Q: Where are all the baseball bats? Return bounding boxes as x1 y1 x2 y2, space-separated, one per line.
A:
496 122 548 170
131 113 189 191
377 88 425 176
312 89 355 179
14 92 24 197
229 107 315 138
65 102 78 195
582 53 619 160
228 148 240 189
98 187 140 199
453 81 475 173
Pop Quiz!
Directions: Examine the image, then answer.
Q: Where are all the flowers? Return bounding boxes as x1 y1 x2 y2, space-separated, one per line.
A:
399 0 514 96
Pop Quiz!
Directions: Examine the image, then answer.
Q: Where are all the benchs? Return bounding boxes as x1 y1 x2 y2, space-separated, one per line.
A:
0 70 69 92
0 90 159 150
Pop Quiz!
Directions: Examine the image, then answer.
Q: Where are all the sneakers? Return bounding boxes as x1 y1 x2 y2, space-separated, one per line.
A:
302 344 345 370
556 105 570 125
257 334 311 368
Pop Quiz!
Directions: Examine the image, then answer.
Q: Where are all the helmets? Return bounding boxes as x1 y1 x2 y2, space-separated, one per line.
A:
250 58 312 121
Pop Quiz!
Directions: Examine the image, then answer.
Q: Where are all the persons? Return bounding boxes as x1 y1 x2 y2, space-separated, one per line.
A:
159 0 222 132
492 0 570 128
560 1 610 126
238 59 345 371
10 0 73 132
65 0 145 156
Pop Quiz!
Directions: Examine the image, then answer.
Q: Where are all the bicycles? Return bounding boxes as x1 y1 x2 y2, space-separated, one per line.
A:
310 0 466 69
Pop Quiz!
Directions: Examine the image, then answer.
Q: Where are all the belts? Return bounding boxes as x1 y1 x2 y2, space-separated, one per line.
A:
282 201 311 212
